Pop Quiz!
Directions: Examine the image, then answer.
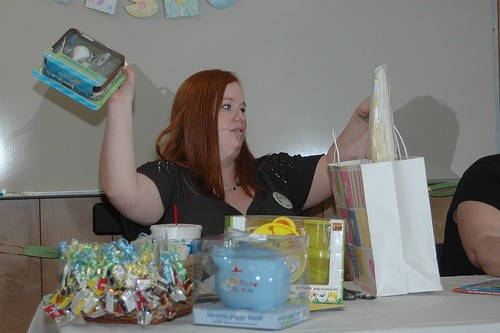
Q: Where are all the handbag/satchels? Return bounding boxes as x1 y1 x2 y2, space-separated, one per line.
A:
327 120 444 296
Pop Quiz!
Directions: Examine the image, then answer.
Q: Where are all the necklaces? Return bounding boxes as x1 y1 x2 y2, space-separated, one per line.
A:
224 179 240 192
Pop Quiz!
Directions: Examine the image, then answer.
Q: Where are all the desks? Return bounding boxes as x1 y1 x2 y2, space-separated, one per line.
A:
24 270 500 333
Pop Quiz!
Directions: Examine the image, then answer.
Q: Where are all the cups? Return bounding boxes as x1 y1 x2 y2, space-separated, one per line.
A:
151 223 204 259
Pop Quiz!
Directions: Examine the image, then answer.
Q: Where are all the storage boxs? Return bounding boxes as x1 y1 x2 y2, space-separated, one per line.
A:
188 233 308 332
33 29 129 110
218 214 348 310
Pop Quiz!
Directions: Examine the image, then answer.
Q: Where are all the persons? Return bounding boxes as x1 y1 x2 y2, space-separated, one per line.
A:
440 153 500 277
99 65 372 237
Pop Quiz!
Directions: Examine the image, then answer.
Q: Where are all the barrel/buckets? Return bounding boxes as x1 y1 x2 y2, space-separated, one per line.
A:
301 220 330 285
